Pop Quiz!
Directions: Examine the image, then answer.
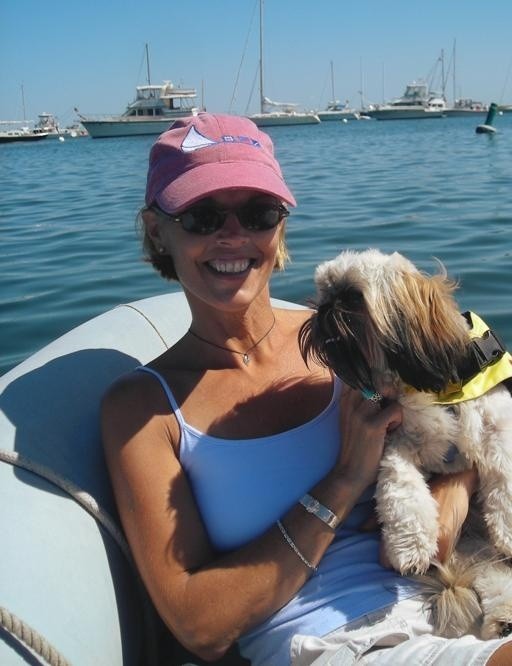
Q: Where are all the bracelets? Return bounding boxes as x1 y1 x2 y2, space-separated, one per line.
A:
297 493 346 532
274 519 319 576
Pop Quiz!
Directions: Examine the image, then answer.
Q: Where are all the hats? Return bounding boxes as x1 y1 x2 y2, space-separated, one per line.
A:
147 111 298 217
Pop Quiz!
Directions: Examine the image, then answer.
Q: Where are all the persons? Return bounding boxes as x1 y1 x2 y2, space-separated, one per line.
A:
99 115 512 666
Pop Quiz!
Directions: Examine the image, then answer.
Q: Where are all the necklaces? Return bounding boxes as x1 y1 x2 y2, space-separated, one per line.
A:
186 311 276 364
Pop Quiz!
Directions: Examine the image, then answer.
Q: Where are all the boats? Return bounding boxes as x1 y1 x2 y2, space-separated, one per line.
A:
0 111 62 141
0 290 317 666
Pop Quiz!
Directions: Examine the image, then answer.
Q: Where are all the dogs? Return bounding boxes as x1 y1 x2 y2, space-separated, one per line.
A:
295 247 512 645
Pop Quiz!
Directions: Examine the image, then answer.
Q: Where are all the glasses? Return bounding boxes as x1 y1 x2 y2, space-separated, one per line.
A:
147 190 291 236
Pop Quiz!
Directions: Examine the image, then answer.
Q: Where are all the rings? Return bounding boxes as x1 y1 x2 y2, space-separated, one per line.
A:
360 386 383 412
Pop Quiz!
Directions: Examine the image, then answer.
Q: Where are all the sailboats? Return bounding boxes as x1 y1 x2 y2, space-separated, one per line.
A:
228 1 321 126
317 61 358 121
358 37 490 120
78 43 205 139
